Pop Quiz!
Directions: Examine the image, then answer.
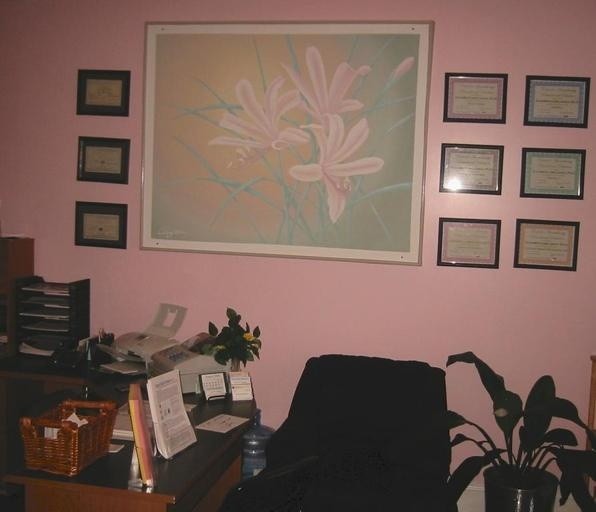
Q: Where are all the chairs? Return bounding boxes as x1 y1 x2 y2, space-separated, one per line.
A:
221 354 458 512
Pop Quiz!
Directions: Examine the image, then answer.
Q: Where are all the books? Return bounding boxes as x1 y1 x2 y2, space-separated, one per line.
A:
113 398 196 442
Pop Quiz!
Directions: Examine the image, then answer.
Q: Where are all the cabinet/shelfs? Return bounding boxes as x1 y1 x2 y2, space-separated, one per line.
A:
15 276 91 361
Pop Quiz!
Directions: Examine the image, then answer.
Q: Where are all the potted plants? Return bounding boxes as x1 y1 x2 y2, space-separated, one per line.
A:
197 306 261 372
446 351 595 512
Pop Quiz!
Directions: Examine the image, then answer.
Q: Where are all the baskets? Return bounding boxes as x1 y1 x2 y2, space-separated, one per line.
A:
18 400 117 477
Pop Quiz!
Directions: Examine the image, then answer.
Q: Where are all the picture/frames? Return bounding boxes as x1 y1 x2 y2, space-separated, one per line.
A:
523 75 590 128
439 143 503 195
76 136 129 184
443 72 508 123
76 70 130 117
513 218 580 271
139 20 435 266
437 218 501 269
520 148 586 200
74 201 127 249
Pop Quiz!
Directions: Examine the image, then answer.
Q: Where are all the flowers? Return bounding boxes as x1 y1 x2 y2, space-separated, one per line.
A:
211 41 386 220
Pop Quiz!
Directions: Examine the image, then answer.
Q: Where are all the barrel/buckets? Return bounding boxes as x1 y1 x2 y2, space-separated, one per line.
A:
242 409 275 483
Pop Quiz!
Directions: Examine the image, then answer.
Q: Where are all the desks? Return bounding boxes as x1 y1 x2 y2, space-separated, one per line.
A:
0 360 257 511
